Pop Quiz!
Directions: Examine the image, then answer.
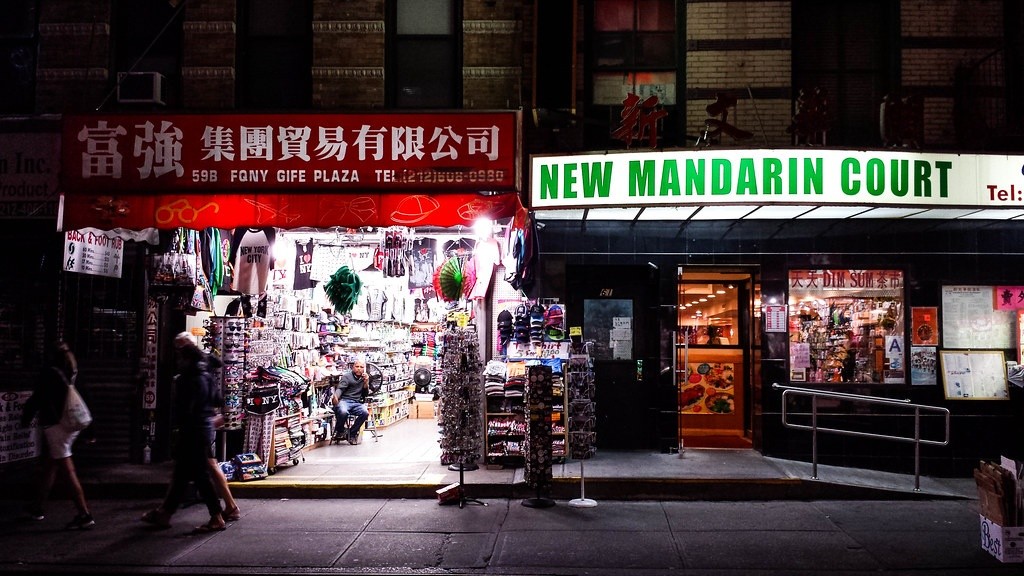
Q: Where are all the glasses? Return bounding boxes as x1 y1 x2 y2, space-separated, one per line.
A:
202 319 253 428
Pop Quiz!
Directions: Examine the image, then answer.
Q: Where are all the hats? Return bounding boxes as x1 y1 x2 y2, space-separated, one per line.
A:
843 330 854 339
497 304 565 346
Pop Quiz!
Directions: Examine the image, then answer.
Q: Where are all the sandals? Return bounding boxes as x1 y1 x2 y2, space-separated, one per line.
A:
195 518 226 533
142 520 170 531
141 512 171 523
224 505 240 521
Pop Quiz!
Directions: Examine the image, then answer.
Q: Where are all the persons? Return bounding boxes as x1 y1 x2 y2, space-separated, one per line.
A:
20 340 95 530
139 331 241 532
706 324 722 344
841 330 857 382
333 360 369 445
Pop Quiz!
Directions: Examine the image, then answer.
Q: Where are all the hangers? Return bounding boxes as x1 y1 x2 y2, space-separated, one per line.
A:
444 227 475 258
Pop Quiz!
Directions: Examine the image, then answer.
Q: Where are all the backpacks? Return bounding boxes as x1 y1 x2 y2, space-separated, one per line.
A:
49 366 92 430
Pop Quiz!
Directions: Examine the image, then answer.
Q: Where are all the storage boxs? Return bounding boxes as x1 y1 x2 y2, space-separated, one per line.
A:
407 393 434 420
980 514 1024 563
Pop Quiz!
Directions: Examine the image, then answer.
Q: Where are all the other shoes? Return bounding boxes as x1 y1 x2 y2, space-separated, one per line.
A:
336 430 347 439
350 428 357 445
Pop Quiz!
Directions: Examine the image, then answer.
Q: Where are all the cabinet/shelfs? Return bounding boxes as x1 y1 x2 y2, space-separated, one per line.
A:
482 364 571 459
268 411 303 472
366 386 409 430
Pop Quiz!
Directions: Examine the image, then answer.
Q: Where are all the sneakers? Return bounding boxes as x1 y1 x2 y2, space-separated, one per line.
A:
29 504 44 521
67 512 95 530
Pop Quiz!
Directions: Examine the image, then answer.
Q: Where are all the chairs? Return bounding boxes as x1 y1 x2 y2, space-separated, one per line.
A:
326 398 357 446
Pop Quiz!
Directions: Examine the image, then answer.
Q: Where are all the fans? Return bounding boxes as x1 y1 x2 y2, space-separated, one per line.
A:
414 368 431 394
364 363 383 396
